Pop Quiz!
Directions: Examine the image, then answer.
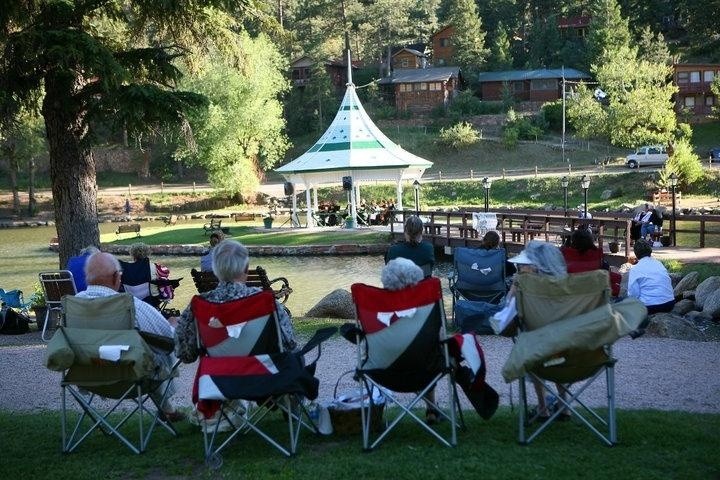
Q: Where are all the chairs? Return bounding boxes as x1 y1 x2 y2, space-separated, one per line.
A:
43 292 179 456
449 247 514 331
39 270 78 342
341 277 467 454
120 258 173 318
191 295 338 469
3 289 31 317
500 269 649 445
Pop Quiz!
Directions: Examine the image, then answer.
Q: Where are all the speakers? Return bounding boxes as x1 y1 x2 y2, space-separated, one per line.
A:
284 182 293 195
343 176 352 190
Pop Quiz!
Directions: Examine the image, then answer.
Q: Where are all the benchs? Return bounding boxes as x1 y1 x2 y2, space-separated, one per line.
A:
191 265 290 305
204 219 222 237
423 217 574 247
650 232 663 244
234 213 256 223
116 224 140 240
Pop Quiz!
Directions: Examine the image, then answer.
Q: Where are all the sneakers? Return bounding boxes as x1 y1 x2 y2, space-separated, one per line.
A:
160 411 185 422
426 409 441 423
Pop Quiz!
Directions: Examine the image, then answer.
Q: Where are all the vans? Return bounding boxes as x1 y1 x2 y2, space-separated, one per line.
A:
626 145 669 170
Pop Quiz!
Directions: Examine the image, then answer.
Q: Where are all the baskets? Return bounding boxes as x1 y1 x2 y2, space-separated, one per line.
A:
328 370 385 433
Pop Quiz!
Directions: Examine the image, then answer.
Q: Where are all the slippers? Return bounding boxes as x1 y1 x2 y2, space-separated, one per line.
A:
529 404 570 421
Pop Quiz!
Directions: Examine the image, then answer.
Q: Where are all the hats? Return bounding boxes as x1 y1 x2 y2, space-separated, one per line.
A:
507 250 532 265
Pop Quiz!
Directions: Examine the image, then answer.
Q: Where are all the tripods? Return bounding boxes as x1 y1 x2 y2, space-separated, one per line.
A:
278 195 302 229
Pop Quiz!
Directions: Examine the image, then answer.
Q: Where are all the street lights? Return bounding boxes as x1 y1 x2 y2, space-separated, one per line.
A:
482 178 493 211
412 180 420 210
561 176 569 215
580 175 591 218
667 173 679 247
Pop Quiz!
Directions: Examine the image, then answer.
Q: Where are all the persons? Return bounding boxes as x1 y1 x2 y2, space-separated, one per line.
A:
173 239 305 423
381 257 445 426
474 230 517 288
314 196 398 228
489 240 574 422
200 231 225 274
629 201 667 245
120 241 152 302
614 237 676 316
383 217 435 276
71 251 186 422
567 227 595 254
